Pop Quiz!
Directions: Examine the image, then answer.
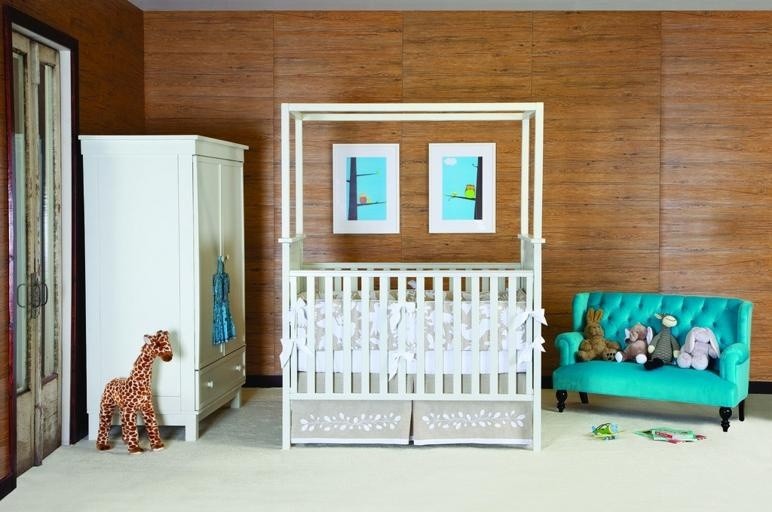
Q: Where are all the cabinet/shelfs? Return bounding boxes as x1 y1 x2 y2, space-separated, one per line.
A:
73 130 252 442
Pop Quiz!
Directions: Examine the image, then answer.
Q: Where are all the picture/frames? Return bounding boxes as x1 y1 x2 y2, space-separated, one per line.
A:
332 140 497 236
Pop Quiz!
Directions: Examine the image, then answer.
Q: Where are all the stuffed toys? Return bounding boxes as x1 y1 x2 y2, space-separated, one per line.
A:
616 321 654 365
577 304 622 362
677 325 722 372
94 329 174 455
644 313 680 371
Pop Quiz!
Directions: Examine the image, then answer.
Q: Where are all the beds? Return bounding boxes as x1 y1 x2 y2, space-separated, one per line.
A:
278 98 546 456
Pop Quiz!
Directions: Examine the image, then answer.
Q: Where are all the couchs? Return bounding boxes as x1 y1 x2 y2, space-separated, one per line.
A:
552 291 755 434
